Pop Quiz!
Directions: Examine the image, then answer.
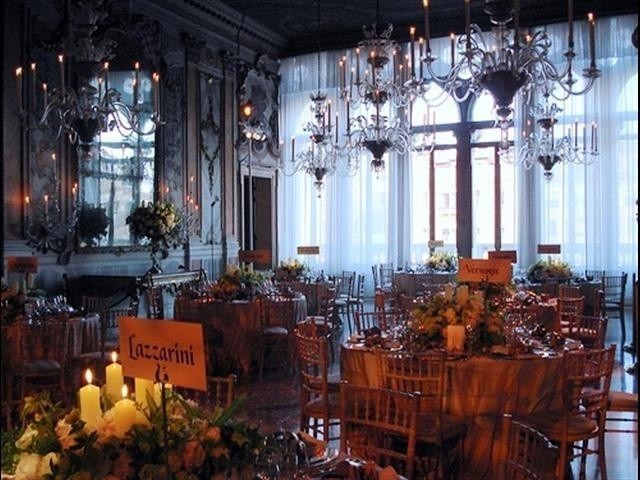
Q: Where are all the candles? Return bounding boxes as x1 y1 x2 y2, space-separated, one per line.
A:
77 366 104 422
114 385 137 436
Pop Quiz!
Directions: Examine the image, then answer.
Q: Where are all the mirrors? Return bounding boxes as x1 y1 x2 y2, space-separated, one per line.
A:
63 15 166 254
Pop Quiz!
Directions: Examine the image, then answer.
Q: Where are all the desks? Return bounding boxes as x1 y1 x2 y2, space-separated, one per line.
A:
337 323 597 420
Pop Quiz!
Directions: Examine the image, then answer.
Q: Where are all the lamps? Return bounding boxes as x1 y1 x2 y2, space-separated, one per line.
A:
420 1 604 161
328 2 438 182
15 1 165 143
21 153 113 255
271 0 366 201
499 1 604 184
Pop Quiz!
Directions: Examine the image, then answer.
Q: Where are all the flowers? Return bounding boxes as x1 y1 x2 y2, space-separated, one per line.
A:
127 200 193 246
0 392 291 480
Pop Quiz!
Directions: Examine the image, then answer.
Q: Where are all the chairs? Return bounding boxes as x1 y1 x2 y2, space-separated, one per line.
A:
2 259 289 408
293 259 640 480
